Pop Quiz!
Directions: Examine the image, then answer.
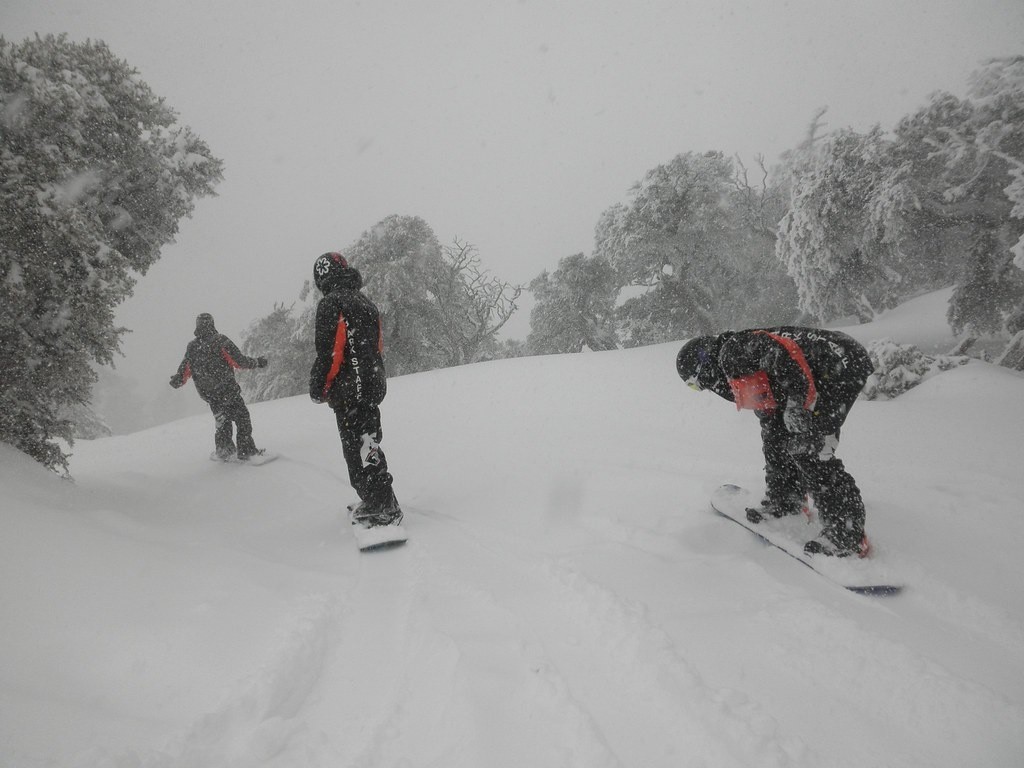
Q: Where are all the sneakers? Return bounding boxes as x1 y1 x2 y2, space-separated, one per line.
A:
217 447 236 459
237 448 266 461
350 494 405 528
804 526 870 559
745 494 812 523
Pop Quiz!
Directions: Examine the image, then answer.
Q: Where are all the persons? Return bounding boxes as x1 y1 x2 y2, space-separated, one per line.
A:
307 253 404 527
169 313 268 460
675 325 875 558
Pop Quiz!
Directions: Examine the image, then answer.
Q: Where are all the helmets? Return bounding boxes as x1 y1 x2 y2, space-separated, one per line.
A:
314 252 348 290
196 313 214 328
675 335 721 393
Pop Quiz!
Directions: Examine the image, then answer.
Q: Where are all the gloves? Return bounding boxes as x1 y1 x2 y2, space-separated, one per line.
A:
170 376 180 389
760 420 780 443
311 397 323 404
257 356 268 368
784 395 814 434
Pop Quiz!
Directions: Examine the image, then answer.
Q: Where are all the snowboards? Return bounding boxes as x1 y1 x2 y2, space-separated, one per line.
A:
346 501 408 554
709 483 906 596
210 449 283 467
